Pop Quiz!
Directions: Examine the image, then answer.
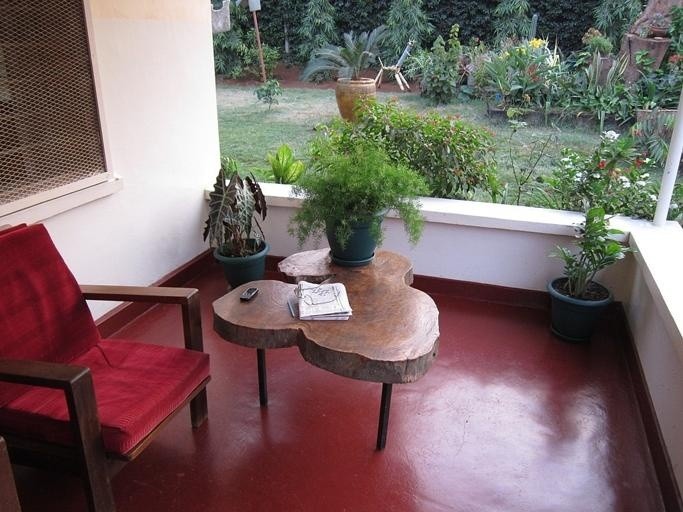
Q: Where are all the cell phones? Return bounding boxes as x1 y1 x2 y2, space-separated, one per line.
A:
240 288 258 300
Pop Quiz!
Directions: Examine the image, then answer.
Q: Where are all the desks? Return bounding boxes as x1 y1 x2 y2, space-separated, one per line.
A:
208 246 442 452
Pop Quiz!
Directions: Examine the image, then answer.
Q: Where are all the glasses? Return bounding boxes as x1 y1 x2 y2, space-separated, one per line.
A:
294 279 339 307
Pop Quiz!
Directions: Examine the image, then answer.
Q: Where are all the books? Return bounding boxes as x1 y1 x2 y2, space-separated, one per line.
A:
296 280 354 324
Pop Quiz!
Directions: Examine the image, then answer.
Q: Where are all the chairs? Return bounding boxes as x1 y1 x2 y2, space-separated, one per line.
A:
0 219 215 512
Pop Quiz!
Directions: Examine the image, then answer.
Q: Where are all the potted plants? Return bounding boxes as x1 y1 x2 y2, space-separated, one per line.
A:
284 135 433 267
543 204 640 346
198 165 271 288
296 24 396 125
